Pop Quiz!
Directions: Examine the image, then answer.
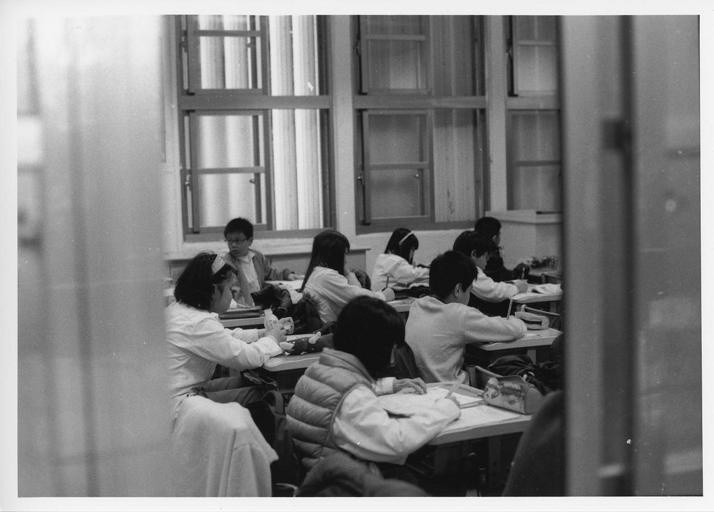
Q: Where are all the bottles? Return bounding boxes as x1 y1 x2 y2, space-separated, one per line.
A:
262 308 279 330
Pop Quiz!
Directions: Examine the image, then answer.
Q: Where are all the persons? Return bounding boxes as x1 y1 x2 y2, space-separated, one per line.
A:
224 218 305 306
405 249 527 383
296 214 529 325
282 295 463 495
169 251 289 453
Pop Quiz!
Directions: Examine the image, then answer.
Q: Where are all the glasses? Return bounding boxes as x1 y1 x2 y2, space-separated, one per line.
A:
225 239 246 244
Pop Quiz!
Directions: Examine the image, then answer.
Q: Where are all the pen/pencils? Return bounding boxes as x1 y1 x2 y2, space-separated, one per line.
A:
386 275 389 289
445 373 465 398
281 327 291 331
521 266 525 279
506 295 512 319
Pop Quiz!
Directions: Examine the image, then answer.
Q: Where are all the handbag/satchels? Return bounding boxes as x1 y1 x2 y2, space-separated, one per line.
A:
488 356 545 396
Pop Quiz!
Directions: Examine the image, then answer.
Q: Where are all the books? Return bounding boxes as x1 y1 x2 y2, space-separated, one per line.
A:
379 387 482 417
528 284 562 294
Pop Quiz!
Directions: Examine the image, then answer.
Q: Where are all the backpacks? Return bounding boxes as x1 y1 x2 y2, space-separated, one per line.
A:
244 390 302 484
